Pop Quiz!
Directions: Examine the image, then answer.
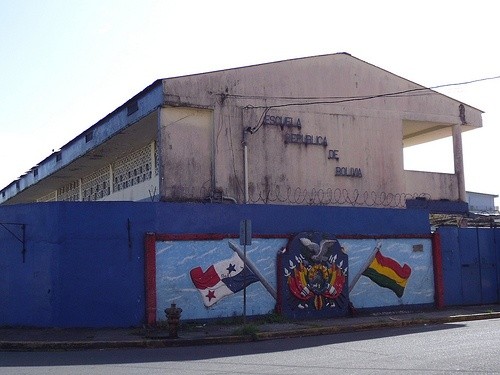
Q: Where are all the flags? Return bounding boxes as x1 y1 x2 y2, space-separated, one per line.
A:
363 253 412 298
190 257 259 308
